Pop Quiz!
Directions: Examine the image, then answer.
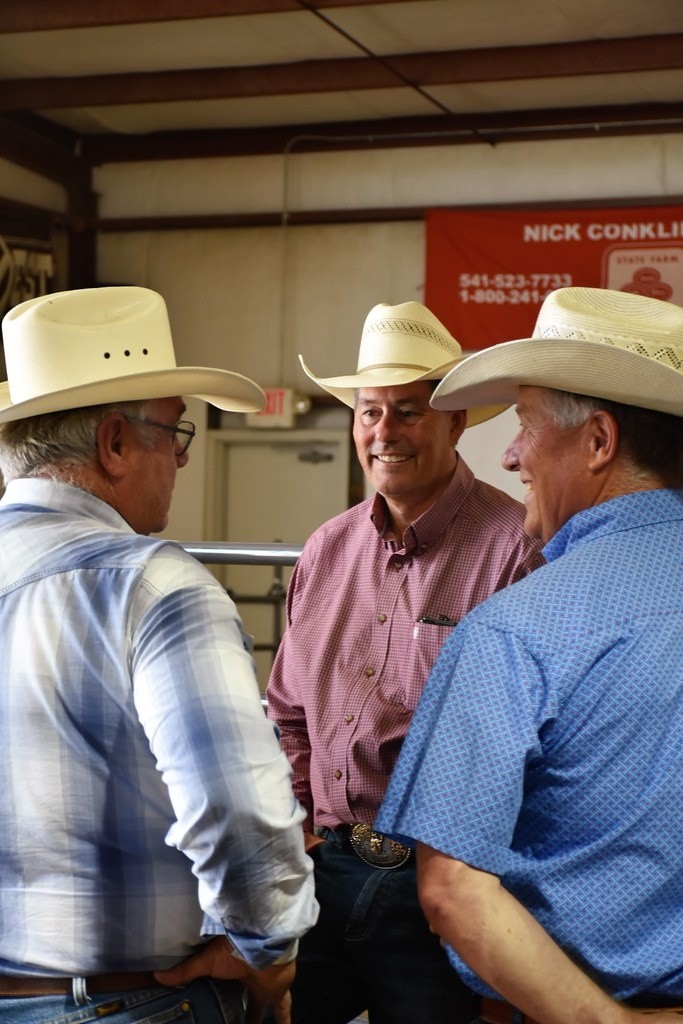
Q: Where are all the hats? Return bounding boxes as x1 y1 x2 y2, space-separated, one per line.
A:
0 284 268 425
430 286 683 419
299 298 516 427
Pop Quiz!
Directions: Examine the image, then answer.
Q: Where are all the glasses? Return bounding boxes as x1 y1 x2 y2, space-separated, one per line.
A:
121 414 196 455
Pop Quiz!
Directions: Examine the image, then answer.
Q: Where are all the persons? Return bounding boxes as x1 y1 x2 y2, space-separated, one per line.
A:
0 286 318 1024
369 286 683 1023
262 300 548 1023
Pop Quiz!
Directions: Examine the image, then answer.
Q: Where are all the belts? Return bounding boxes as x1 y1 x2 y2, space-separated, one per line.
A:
315 818 418 869
477 996 537 1023
0 970 156 999
